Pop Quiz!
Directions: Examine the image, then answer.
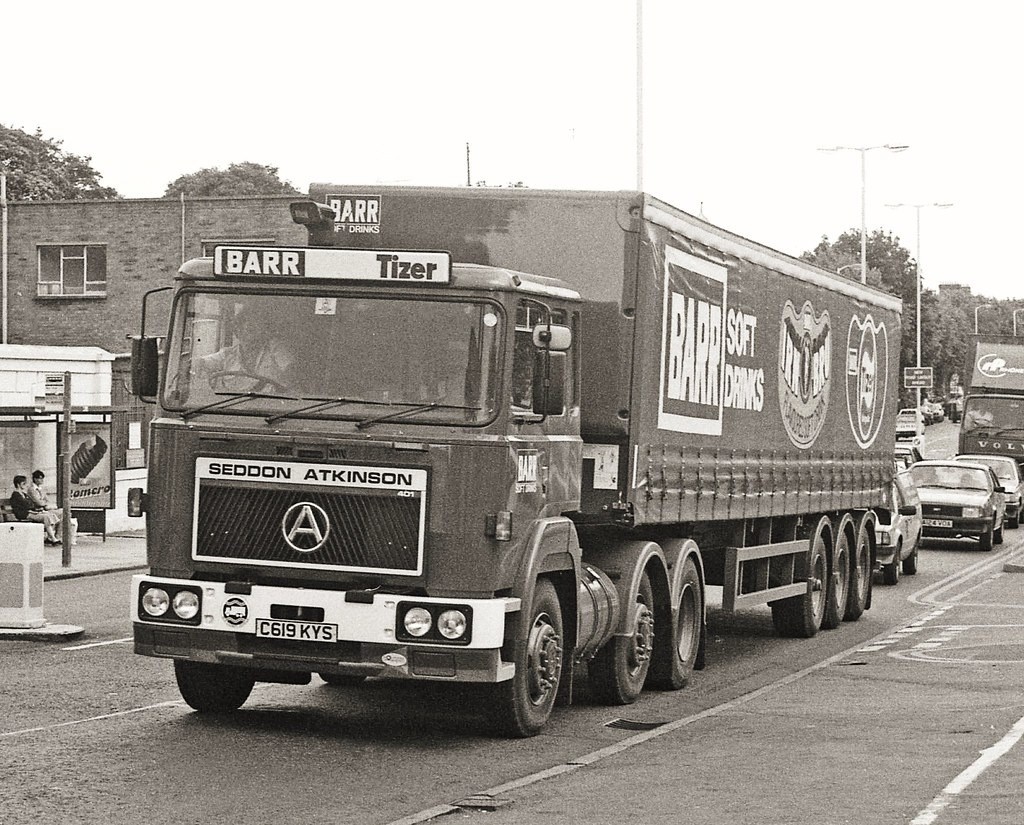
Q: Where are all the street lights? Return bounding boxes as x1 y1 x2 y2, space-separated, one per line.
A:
883 198 958 439
819 141 911 285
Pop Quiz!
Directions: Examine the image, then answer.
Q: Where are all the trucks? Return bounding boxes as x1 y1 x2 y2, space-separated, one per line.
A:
126 185 903 742
955 334 1024 480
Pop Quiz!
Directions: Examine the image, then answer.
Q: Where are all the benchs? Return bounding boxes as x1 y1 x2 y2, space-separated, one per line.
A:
0 498 47 536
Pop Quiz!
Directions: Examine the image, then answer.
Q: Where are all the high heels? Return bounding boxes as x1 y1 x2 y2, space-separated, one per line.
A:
53 540 64 547
44 538 53 545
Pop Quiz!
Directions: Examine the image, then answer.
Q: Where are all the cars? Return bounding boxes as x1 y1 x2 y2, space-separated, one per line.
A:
874 400 1024 589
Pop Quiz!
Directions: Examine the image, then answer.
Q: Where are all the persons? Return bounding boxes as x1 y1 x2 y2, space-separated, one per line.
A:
190 304 306 396
28 471 72 544
10 475 62 547
